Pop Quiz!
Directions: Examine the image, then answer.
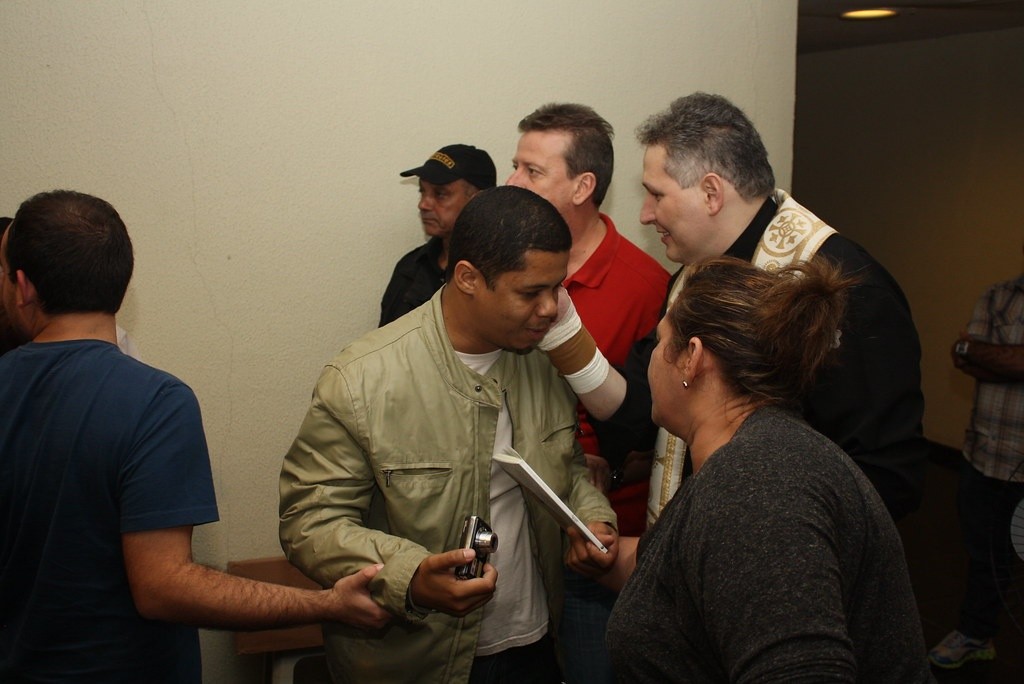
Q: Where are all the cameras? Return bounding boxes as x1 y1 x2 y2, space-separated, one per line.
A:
454 514 498 581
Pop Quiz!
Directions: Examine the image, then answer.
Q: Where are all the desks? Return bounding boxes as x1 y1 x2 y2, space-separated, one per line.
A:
227 557 325 684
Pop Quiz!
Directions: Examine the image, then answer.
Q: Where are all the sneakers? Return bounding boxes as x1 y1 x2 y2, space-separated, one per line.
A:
928 629 996 668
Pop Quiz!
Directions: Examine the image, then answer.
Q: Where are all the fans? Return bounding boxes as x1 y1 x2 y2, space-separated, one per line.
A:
988 460 1024 642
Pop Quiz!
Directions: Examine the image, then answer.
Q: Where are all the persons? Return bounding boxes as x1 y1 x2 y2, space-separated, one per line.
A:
279 184 620 684
505 102 671 684
0 188 393 684
532 91 926 529
929 276 1024 668
593 254 934 684
378 144 497 332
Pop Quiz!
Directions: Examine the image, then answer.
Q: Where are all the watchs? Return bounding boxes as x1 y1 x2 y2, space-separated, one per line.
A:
609 459 625 488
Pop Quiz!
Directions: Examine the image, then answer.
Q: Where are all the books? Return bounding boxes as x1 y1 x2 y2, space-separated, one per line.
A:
492 445 608 554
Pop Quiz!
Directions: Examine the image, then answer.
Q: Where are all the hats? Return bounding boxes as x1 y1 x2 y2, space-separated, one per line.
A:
400 145 496 191
